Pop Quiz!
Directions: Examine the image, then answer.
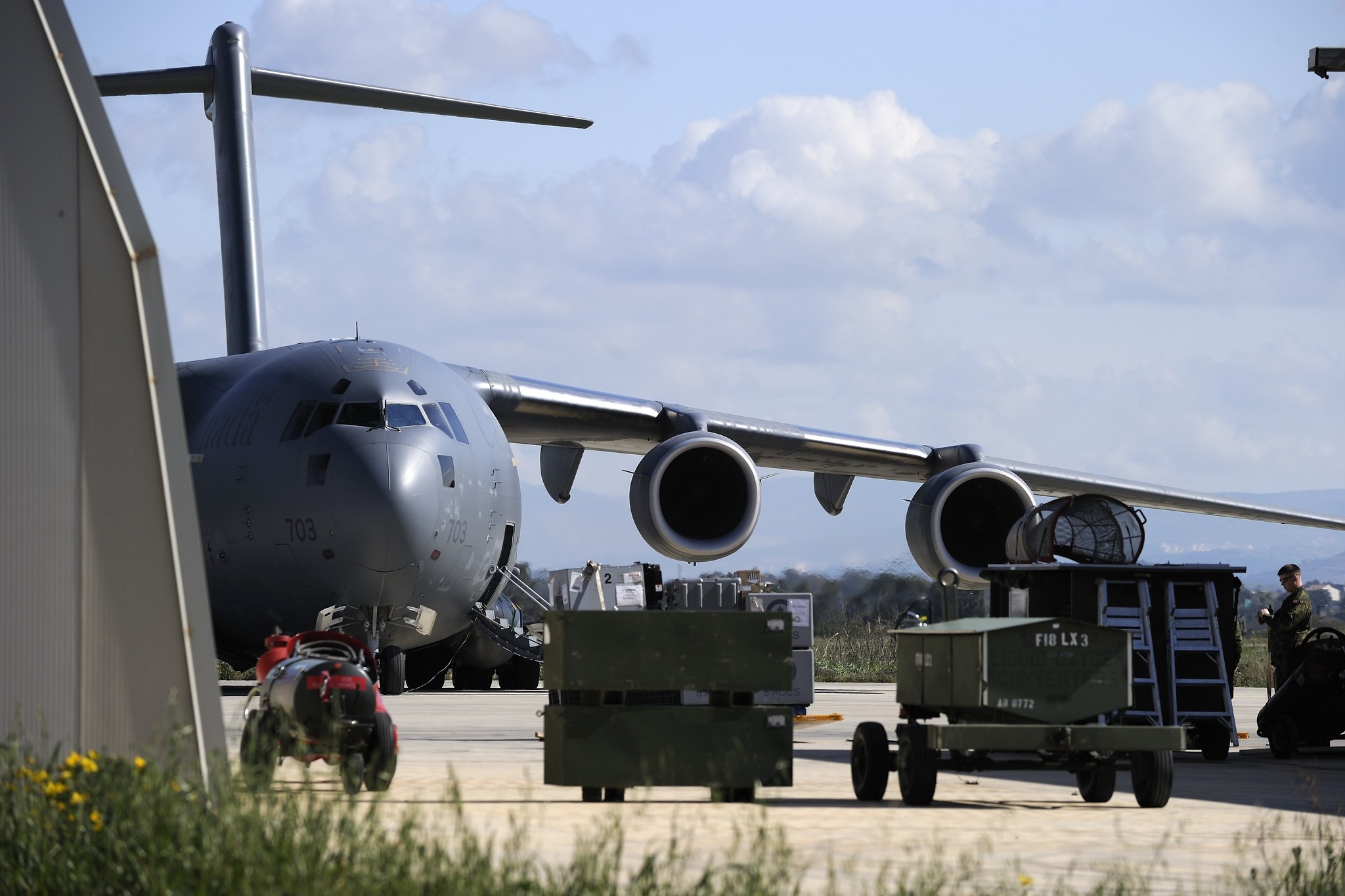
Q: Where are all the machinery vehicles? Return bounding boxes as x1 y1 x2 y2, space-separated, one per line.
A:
239 626 398 796
979 493 1248 761
850 567 1185 809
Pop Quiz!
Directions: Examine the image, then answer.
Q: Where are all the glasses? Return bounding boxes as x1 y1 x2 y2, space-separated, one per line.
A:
1279 574 1298 583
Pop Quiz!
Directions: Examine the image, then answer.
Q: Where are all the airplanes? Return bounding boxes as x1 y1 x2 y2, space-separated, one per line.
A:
94 16 1345 689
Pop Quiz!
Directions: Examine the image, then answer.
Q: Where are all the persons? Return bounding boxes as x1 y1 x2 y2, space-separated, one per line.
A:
1257 564 1312 694
